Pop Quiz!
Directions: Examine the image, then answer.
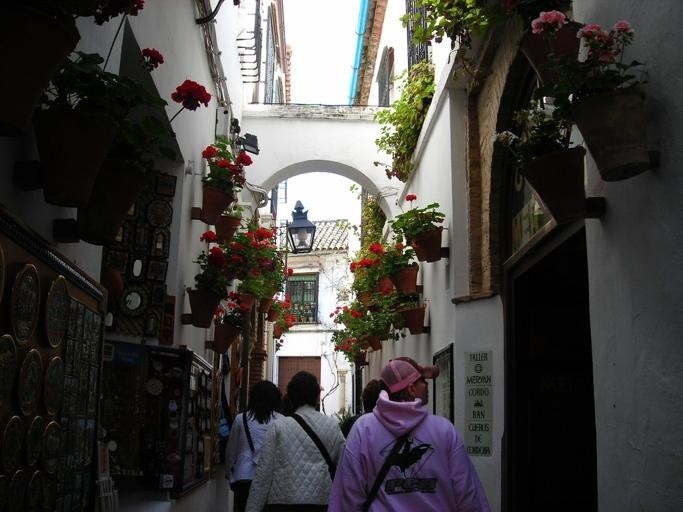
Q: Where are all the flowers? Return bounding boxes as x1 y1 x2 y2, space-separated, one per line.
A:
329 193 445 366
144 47 213 121
530 11 656 100
183 142 294 328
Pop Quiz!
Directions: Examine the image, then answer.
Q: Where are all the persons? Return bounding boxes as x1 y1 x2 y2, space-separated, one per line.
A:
244 371 349 511
326 356 492 512
223 379 286 511
340 378 390 440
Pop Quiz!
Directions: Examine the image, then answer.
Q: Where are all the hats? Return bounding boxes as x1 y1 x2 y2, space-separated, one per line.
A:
380 357 439 393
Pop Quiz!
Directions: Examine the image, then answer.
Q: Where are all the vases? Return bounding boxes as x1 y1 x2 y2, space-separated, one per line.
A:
566 93 653 183
273 326 281 337
214 327 238 353
509 28 579 80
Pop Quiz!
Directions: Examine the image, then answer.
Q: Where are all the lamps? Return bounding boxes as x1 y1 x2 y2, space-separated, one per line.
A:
285 200 315 254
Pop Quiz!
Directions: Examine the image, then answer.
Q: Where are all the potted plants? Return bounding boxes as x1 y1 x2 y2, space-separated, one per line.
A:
491 104 585 223
